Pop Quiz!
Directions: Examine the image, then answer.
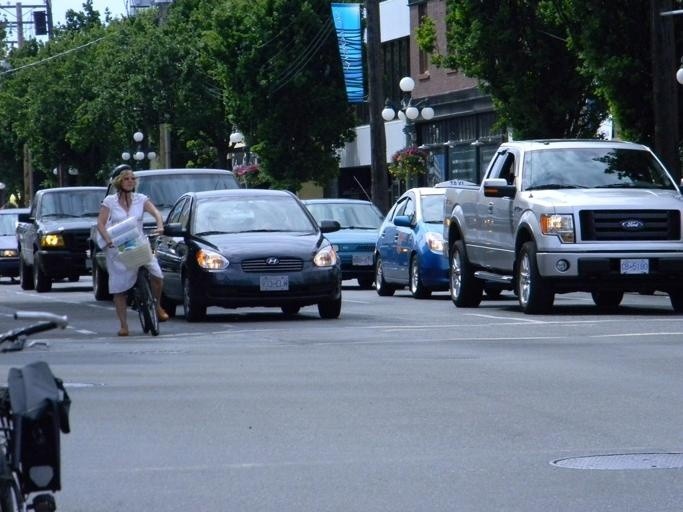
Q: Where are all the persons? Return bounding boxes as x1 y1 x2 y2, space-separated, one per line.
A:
96 164 170 336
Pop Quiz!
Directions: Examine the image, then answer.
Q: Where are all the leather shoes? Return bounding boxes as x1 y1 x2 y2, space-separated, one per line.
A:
155 307 169 321
118 327 128 336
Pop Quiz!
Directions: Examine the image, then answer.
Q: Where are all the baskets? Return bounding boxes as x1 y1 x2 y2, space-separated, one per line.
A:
116 235 152 269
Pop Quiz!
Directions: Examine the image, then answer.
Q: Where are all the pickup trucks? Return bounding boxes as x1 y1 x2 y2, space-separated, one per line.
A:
444 140 683 316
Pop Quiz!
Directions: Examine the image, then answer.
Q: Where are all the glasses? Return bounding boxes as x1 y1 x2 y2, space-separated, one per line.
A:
123 177 137 180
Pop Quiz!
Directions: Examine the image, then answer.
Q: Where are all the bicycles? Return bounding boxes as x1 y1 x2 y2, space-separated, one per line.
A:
100 228 163 336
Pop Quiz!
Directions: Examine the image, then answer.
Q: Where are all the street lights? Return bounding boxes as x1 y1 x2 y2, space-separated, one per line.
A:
121 131 157 170
226 126 246 165
380 77 434 186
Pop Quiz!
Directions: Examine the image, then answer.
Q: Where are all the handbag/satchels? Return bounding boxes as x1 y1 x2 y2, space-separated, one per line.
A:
90 223 106 244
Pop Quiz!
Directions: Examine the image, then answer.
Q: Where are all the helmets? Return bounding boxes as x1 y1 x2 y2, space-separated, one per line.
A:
111 164 132 180
